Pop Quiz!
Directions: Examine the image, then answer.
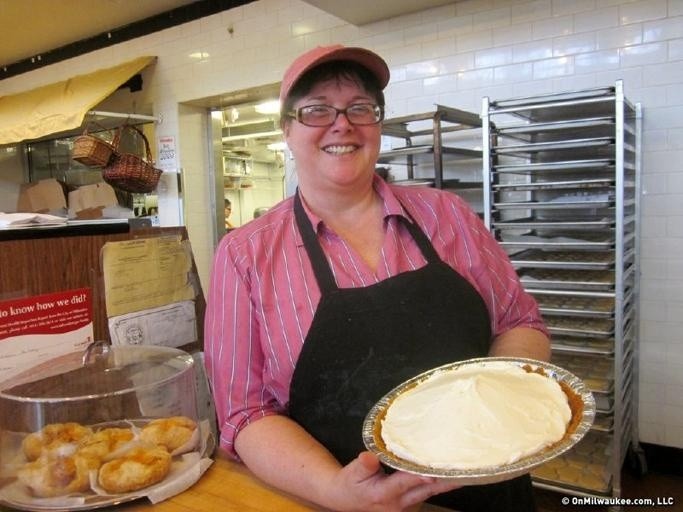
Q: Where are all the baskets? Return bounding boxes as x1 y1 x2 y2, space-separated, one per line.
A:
102 123 162 193
72 121 113 167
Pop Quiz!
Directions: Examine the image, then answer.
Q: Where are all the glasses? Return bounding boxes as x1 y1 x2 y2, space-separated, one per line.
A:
283 103 385 128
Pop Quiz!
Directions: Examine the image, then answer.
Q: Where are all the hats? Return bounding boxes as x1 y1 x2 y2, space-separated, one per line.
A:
278 44 391 110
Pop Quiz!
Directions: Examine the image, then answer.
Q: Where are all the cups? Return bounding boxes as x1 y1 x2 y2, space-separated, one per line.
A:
132 193 159 220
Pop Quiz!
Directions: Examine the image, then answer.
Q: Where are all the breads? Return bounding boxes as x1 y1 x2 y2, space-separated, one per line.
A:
22 415 198 498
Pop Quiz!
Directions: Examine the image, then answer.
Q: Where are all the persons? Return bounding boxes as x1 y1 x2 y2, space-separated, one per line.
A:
199 41 552 512
224 198 233 228
